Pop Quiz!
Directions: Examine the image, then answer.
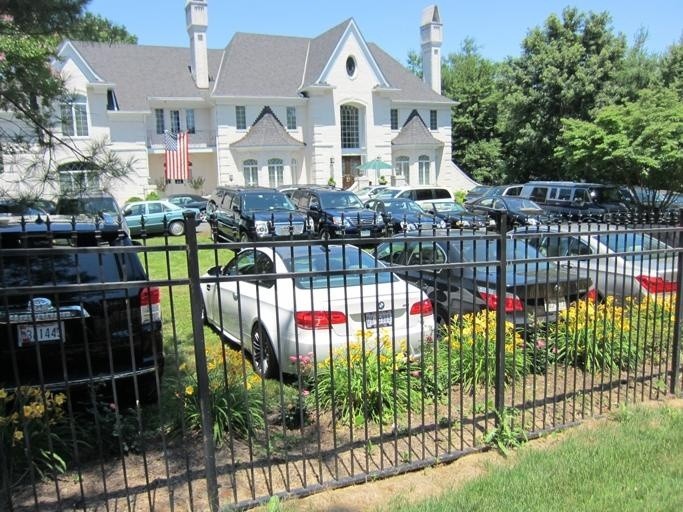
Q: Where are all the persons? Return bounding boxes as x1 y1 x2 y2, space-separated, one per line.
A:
377 175 388 186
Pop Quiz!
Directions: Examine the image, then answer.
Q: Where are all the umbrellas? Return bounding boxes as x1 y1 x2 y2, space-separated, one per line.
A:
353 156 396 185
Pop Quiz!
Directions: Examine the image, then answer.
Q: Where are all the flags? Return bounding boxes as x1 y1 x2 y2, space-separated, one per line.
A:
164 128 188 181
